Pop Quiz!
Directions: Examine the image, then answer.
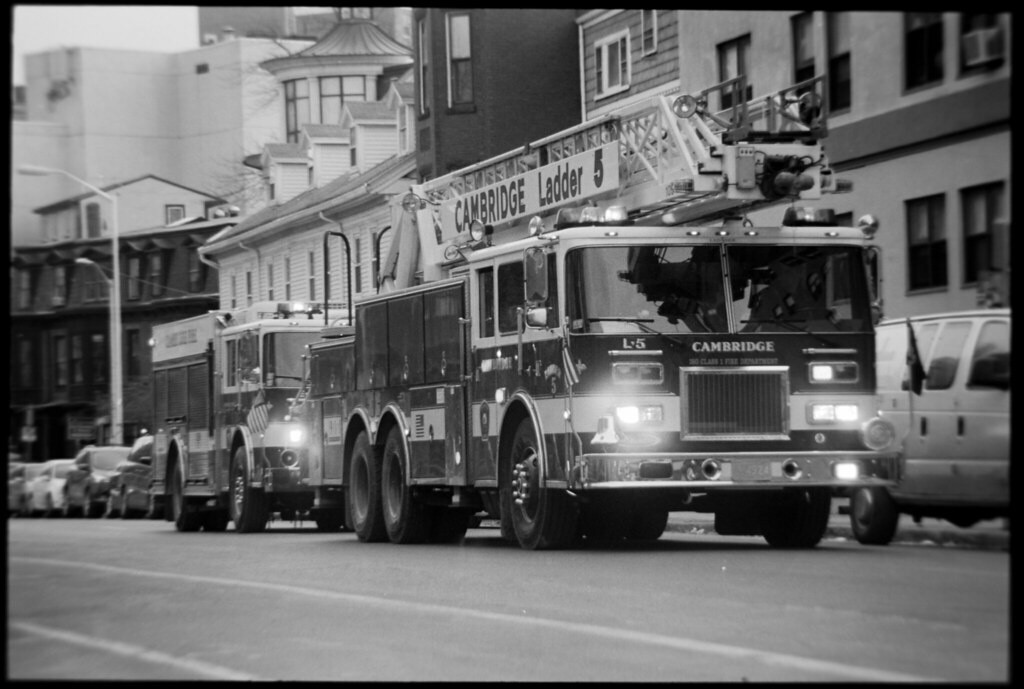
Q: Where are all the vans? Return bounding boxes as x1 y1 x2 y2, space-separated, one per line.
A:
832 305 1013 546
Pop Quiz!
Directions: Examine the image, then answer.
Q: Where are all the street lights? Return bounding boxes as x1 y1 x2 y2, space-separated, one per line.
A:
74 254 115 444
18 165 123 446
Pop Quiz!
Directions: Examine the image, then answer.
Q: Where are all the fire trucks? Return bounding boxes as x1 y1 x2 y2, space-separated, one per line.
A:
293 67 905 553
146 297 345 534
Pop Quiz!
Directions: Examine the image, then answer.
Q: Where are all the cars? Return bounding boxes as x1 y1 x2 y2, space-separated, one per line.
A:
8 431 154 519
